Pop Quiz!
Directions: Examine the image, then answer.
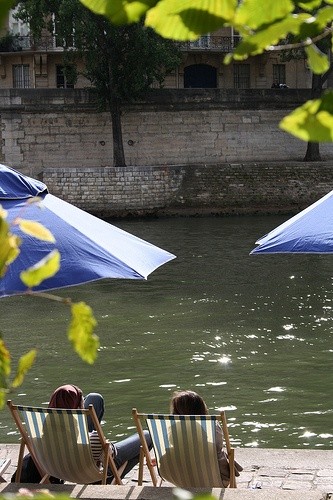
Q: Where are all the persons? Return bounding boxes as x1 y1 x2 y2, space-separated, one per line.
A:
158 390 230 488
42 384 153 484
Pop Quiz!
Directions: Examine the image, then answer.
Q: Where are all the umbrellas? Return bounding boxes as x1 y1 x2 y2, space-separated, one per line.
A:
0 160 177 302
249 187 331 254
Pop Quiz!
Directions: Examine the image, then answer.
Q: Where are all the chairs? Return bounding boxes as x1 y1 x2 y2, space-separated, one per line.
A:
6 399 128 484
131 408 244 488
